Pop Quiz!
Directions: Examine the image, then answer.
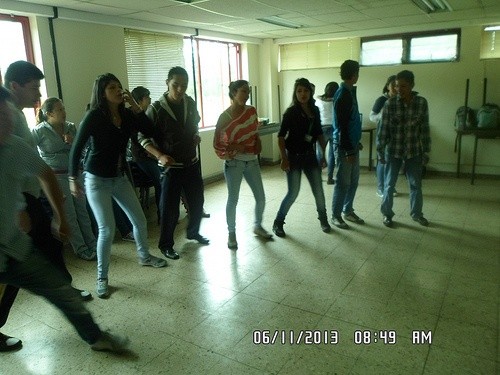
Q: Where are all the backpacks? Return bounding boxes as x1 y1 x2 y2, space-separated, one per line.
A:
453 105 474 131
476 106 499 129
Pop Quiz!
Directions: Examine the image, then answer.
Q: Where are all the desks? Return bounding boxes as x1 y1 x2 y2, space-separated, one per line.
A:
361 126 376 171
454 129 500 185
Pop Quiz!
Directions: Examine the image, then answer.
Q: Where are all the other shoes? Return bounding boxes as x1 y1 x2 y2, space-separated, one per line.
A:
139 254 167 267
122 231 135 242
91 330 130 351
393 191 400 197
375 191 384 198
76 249 97 261
327 179 336 187
330 214 348 229
383 215 392 227
160 248 180 259
343 212 364 224
187 233 209 243
254 226 273 238
227 233 238 248
412 216 429 226
71 286 92 300
0 332 22 352
96 279 110 299
271 220 286 237
317 215 331 232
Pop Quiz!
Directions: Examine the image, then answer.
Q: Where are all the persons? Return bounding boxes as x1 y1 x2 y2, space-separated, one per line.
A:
80 86 210 242
213 80 273 248
289 82 340 185
375 69 432 228
330 60 365 229
0 87 130 351
273 78 330 237
68 73 167 299
369 75 400 196
0 61 93 351
31 96 97 259
138 67 209 259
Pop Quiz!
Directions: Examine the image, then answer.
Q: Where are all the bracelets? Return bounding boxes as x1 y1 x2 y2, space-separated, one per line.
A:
157 153 163 159
68 177 78 180
